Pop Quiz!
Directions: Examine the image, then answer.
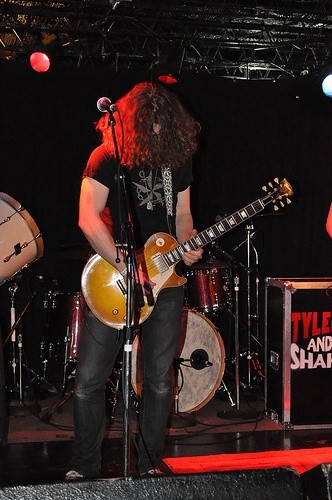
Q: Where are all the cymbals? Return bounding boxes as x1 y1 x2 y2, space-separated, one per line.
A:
177 257 234 269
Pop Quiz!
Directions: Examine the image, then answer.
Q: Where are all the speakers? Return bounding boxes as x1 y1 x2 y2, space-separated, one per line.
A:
0 466 311 499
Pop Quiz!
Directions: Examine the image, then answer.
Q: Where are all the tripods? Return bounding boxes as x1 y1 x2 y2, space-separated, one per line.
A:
0 224 265 405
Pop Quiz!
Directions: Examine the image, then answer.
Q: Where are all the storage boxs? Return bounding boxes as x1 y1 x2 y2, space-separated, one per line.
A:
262 276 332 430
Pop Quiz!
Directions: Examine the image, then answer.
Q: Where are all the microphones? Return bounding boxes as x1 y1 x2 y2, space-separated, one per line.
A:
96 97 117 113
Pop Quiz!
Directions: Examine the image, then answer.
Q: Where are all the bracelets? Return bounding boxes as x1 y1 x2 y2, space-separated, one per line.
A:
120 268 127 277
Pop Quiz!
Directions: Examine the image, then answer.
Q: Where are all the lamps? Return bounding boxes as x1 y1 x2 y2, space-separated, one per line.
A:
157 43 186 84
29 40 63 73
319 64 332 98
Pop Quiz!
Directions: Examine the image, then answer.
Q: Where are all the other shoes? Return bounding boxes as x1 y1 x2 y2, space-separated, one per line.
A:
144 469 165 476
66 470 85 481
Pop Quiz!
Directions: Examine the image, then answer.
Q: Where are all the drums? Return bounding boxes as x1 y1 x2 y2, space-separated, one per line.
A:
122 307 226 415
0 191 45 286
66 292 90 358
180 268 232 313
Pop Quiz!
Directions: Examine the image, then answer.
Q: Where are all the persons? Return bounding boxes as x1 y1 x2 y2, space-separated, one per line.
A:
64 82 204 482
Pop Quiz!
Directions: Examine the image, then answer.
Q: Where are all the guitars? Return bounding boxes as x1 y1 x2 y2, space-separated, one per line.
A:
79 176 294 333
